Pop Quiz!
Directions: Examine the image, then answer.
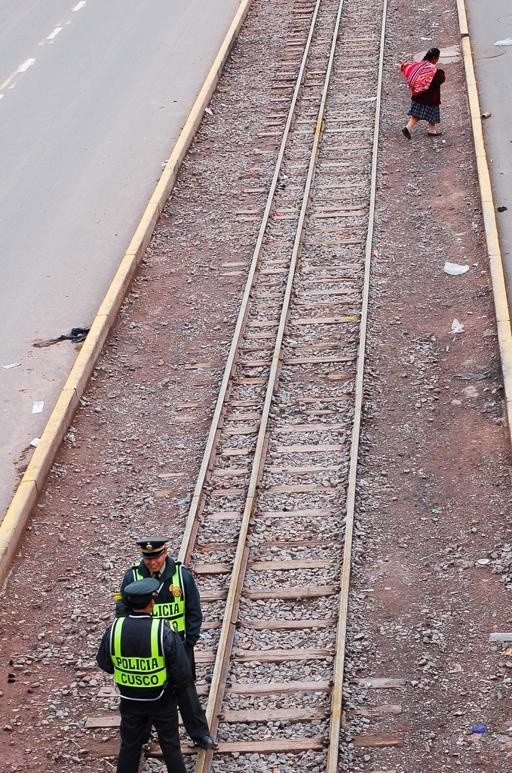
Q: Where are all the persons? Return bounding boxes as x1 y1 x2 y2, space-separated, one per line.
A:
96 578 192 772
402 45 447 140
112 533 219 752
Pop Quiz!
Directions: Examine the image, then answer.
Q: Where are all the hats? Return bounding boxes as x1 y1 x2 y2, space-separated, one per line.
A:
135 536 170 559
122 576 161 605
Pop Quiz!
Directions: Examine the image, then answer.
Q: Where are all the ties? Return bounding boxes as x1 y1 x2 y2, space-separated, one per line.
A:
152 571 160 580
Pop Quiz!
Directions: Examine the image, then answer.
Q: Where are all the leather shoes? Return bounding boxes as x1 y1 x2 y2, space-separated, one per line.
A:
196 735 217 749
402 126 411 140
428 131 442 137
